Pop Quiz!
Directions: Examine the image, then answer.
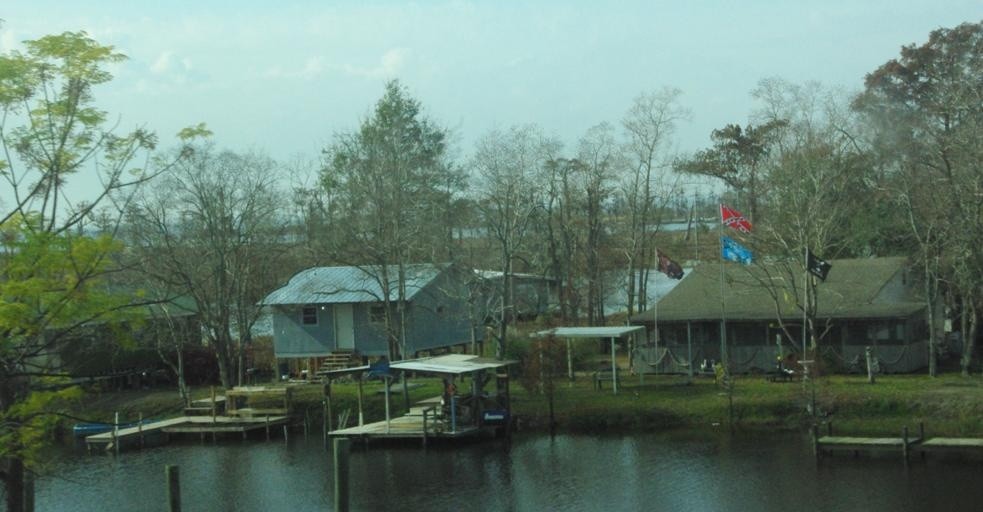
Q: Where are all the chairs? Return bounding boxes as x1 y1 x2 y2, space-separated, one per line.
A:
592 361 622 392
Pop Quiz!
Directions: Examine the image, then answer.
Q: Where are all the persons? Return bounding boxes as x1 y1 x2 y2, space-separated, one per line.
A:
711 359 728 390
443 378 457 396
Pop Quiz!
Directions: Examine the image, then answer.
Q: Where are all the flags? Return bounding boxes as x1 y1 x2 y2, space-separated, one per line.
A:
655 247 683 280
802 246 832 281
720 204 752 235
721 233 753 266
